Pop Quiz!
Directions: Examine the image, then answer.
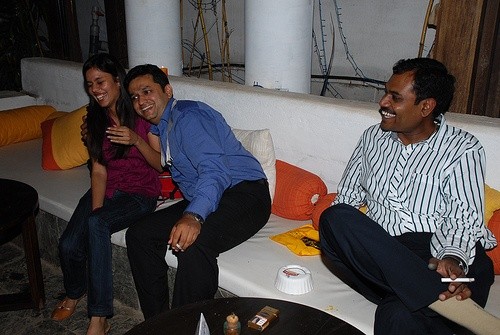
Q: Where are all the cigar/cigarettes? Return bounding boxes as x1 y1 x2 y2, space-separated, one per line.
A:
441 277 474 282
176 244 181 249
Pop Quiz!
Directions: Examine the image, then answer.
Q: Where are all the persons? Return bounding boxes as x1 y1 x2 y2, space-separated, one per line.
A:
51 55 164 335
319 58 500 335
81 64 272 335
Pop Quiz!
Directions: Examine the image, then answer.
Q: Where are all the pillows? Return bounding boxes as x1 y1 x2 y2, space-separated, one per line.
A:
230 128 338 231
483 184 500 276
0 102 92 172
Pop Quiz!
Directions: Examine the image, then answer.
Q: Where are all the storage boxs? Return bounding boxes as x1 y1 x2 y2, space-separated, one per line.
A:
247 306 280 332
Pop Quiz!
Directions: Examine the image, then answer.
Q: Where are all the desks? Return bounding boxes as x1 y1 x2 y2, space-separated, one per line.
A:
124 297 367 335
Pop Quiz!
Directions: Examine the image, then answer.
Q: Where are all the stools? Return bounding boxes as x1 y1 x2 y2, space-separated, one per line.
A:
0 177 48 314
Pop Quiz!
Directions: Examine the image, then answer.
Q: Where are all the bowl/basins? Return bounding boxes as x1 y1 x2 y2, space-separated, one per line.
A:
275 264 313 295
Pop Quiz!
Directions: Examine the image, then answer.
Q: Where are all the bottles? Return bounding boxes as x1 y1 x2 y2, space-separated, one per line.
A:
224 312 241 335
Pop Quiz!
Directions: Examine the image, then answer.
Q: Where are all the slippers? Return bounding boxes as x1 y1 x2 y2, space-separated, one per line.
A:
51 297 78 321
104 323 111 335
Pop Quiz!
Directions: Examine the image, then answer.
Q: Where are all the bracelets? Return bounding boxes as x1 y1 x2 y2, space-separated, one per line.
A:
185 212 204 224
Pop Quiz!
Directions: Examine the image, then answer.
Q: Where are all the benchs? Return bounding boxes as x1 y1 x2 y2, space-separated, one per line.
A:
0 56 500 335
